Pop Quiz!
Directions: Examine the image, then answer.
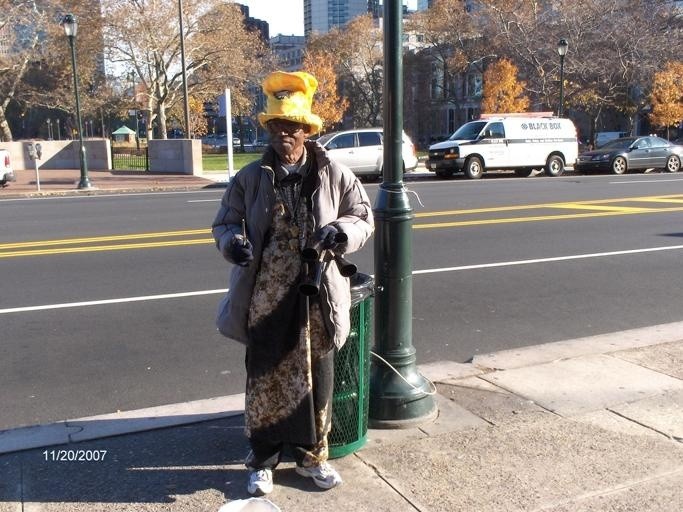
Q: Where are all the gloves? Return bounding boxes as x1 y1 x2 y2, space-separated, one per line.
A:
232 239 253 267
321 226 340 251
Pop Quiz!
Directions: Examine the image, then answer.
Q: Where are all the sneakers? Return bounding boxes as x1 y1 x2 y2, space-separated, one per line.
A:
295 462 342 490
247 469 275 496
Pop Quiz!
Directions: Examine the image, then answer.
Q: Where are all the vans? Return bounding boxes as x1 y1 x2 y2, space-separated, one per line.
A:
424 110 580 181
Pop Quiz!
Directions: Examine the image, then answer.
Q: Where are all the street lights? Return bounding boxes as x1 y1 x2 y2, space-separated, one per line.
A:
556 37 570 119
128 69 143 151
44 115 96 141
57 11 92 189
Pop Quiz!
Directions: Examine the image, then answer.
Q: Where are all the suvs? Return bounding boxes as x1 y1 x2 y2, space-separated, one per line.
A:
316 126 420 183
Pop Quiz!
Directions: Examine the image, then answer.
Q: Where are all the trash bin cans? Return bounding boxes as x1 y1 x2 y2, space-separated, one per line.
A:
329 271 375 460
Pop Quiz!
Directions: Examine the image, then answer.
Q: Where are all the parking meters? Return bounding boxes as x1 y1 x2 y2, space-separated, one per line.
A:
26 142 43 194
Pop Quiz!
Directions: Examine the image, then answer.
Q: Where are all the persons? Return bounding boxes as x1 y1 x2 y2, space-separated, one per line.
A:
211 120 377 495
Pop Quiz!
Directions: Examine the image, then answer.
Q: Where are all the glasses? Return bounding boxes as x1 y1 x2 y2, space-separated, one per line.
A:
266 120 303 134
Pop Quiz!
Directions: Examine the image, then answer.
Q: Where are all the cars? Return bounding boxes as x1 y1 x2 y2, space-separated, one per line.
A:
572 134 683 176
201 132 264 148
0 149 14 188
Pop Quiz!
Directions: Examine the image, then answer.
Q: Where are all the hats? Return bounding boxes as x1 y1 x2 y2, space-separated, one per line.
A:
258 71 321 135
